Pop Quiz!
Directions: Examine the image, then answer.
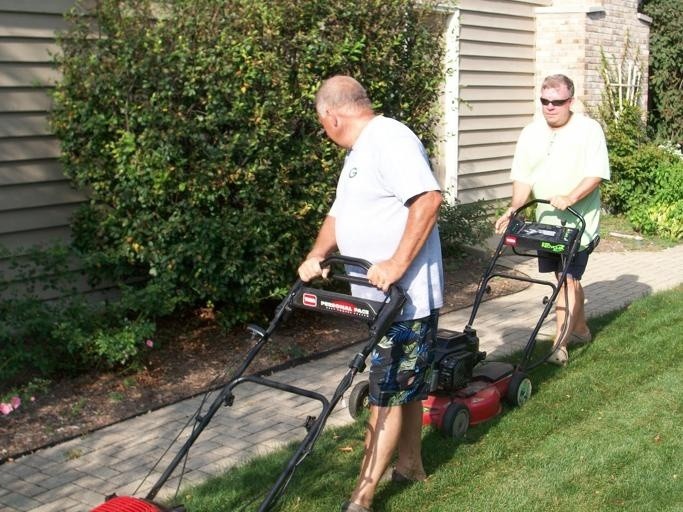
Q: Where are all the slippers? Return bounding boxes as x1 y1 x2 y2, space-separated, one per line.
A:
547 346 567 364
380 466 410 482
568 332 591 344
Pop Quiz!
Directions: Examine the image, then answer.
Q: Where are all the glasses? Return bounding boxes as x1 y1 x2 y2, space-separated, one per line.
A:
540 97 570 106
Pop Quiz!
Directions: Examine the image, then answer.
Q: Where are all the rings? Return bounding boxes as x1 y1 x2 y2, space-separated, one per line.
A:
378 281 384 285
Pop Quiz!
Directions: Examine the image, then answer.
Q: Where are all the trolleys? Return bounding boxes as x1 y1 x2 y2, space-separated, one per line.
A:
81 258 412 511
351 199 587 441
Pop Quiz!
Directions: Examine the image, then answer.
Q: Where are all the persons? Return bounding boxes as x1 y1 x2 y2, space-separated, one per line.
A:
296 74 447 512
493 73 611 368
